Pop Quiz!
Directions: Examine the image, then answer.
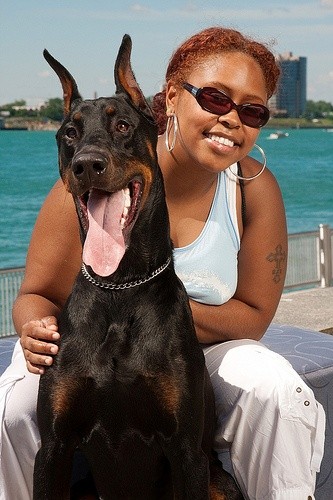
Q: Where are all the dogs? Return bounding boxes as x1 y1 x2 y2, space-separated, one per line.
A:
32 34 246 500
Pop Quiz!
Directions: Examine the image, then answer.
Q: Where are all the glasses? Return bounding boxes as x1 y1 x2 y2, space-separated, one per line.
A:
182 81 271 128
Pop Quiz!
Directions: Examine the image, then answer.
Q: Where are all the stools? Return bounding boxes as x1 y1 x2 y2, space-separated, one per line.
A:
0 320 333 500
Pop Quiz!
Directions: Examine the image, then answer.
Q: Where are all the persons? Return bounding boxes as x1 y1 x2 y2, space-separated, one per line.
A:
1 26 325 500
